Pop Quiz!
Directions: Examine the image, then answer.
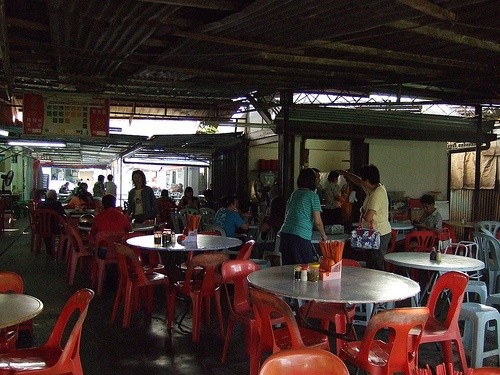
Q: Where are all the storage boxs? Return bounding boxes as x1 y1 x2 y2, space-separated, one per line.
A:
325 224 344 235
410 208 423 221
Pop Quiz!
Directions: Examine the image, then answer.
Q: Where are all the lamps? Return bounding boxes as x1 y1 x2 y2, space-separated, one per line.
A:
5 138 67 148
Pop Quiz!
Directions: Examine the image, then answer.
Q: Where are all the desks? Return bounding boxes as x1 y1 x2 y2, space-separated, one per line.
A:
77 223 154 232
352 221 414 230
0 294 43 328
443 220 495 256
57 193 71 199
248 223 259 228
62 209 95 217
384 251 485 351
311 231 347 257
247 264 422 355
126 234 242 328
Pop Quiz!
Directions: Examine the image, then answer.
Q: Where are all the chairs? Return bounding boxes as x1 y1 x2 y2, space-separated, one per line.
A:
0 191 500 375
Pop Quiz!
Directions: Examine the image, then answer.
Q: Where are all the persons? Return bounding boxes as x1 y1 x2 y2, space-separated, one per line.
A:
156 182 256 259
409 195 443 281
279 168 329 316
333 163 391 313
127 170 158 267
311 167 366 235
89 195 133 288
30 183 67 252
259 186 287 266
93 174 117 216
59 179 94 209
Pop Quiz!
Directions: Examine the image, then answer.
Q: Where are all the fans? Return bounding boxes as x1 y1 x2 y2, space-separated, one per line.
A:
0 170 14 193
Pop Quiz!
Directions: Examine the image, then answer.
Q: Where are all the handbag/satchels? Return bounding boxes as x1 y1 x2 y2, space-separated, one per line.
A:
350 212 380 250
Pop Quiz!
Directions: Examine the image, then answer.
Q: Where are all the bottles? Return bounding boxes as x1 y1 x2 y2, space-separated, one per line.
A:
308 263 321 282
153 228 176 247
461 217 467 224
436 250 442 261
300 264 309 281
430 247 436 260
293 266 302 280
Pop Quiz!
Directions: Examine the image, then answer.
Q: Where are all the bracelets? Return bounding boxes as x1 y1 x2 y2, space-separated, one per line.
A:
344 170 348 176
